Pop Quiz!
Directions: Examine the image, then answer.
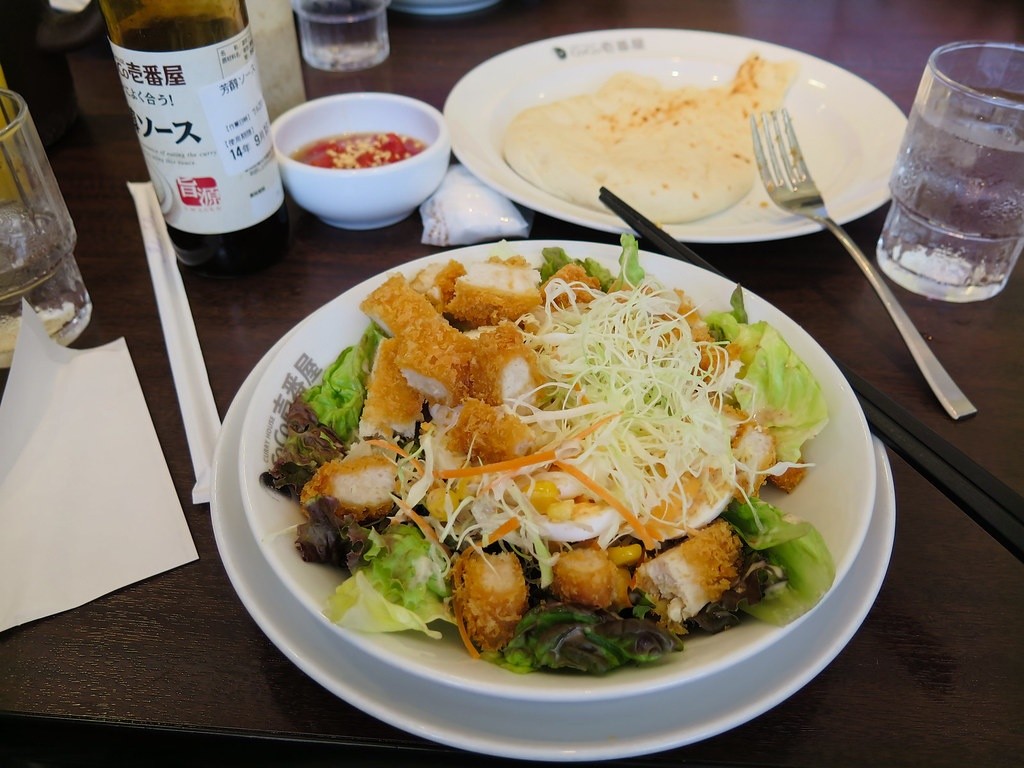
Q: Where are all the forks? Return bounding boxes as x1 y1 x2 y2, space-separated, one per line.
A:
748 107 979 420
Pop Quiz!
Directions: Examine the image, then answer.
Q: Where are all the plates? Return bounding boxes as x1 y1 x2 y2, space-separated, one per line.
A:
237 241 876 703
442 27 910 242
210 240 896 762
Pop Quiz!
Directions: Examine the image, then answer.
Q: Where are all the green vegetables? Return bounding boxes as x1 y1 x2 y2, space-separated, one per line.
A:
258 231 838 676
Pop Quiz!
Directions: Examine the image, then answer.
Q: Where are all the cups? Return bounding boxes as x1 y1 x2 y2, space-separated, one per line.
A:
0 89 93 371
875 41 1024 303
292 0 392 71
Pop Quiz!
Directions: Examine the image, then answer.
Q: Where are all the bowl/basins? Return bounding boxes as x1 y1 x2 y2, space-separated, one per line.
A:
270 92 452 230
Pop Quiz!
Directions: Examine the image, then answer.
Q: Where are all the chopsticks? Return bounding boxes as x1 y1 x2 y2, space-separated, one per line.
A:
600 185 1024 565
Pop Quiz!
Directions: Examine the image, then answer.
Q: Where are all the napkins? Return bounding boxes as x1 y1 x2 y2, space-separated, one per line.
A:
0 295 198 633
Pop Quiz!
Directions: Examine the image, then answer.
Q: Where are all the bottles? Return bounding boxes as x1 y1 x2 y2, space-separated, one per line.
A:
99 0 292 284
